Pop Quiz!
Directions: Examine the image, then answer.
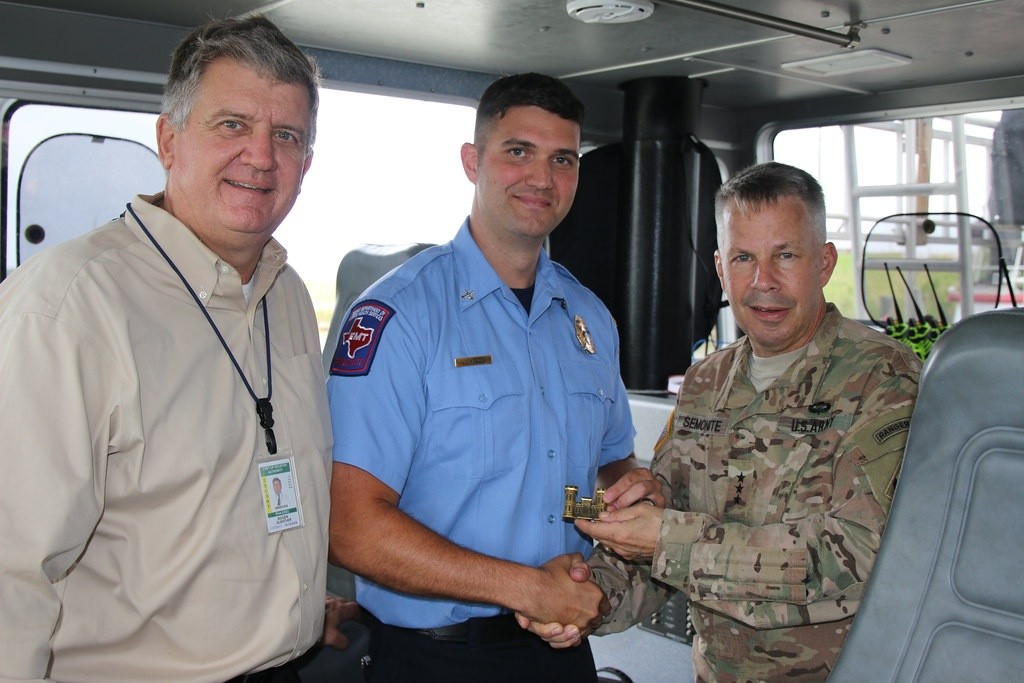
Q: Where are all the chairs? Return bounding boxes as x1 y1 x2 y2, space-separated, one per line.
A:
823 303 1024 683
319 241 443 602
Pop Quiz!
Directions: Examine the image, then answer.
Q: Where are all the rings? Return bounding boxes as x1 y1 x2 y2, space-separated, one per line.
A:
642 498 656 506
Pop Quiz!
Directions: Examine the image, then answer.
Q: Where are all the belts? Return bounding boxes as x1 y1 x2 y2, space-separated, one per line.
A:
418 612 540 641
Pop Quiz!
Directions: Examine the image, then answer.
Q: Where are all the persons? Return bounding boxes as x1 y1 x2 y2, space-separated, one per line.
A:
971 107 1024 289
326 73 664 683
0 12 336 683
516 161 926 683
